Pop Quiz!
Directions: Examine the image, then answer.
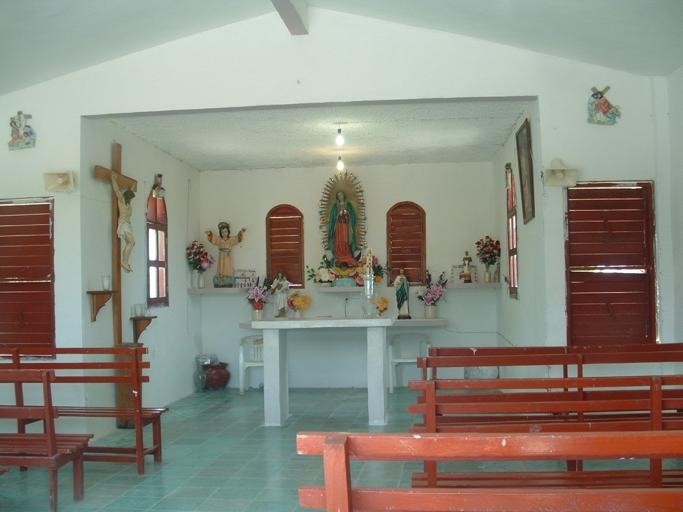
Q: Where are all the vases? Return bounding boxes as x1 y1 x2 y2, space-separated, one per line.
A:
423 305 438 319
375 310 382 317
484 272 491 283
294 309 302 319
191 271 206 289
255 308 263 320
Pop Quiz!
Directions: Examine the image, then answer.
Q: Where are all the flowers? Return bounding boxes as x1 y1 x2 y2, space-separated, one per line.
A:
373 296 389 313
474 235 501 271
286 292 312 311
414 271 450 305
246 281 272 310
185 240 214 270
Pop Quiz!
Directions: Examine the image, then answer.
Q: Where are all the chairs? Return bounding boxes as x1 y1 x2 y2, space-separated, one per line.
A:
239 334 266 396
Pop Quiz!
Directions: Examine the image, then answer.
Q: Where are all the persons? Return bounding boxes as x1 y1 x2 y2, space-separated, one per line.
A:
109 172 137 273
326 191 359 266
204 221 246 288
391 267 411 318
268 270 293 318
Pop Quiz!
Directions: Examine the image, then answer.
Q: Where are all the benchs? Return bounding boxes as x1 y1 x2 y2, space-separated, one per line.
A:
0 343 169 511
292 342 683 512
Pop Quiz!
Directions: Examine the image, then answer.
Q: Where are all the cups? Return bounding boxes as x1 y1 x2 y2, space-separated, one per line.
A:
102 276 110 291
135 304 145 317
